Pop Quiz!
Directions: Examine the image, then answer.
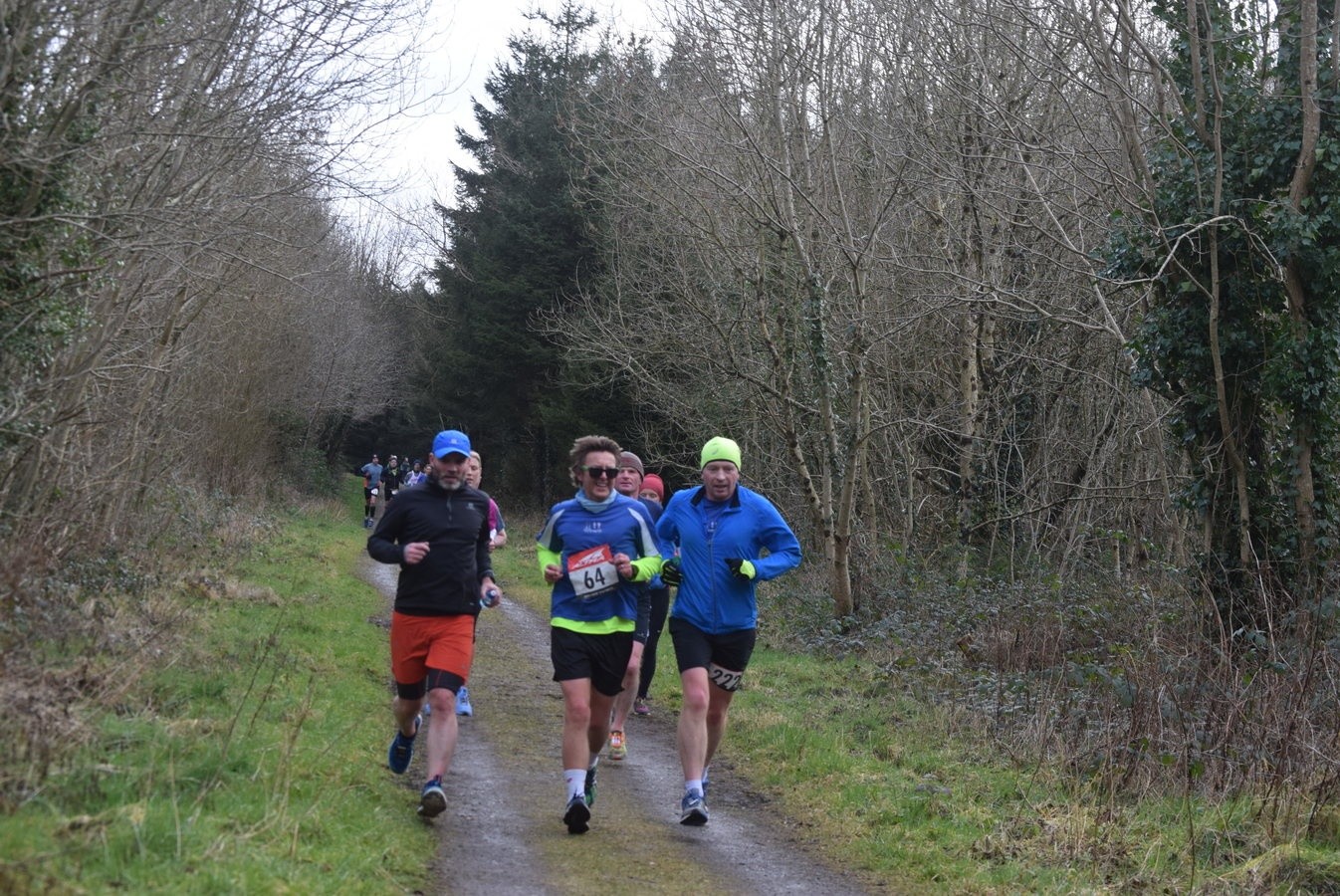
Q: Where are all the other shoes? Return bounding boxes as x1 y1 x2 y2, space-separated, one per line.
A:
364 518 374 527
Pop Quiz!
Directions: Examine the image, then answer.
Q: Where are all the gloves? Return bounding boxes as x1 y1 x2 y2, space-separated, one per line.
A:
725 557 757 582
659 559 683 586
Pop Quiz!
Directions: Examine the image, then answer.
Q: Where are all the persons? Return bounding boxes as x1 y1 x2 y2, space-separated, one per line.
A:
361 454 431 527
424 452 508 715
366 429 504 816
605 450 665 761
654 436 803 825
537 436 662 835
634 472 670 717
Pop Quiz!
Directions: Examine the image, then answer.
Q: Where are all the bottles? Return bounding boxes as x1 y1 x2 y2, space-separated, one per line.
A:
479 590 498 610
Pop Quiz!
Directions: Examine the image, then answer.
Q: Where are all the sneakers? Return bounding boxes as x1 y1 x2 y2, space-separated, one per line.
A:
424 704 431 716
418 775 447 817
607 730 626 759
584 766 597 809
455 686 472 716
680 782 710 825
564 797 591 834
633 697 650 716
388 714 422 775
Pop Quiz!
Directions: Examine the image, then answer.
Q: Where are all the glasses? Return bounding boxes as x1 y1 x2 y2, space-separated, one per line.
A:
579 465 619 480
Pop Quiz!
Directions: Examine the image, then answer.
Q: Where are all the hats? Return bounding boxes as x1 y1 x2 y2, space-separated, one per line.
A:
639 473 663 499
432 430 470 459
701 436 741 471
388 455 397 461
414 460 421 464
372 454 379 458
620 451 644 481
